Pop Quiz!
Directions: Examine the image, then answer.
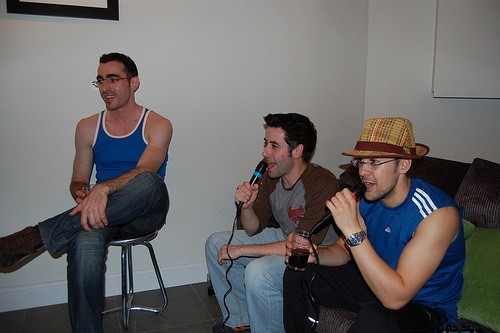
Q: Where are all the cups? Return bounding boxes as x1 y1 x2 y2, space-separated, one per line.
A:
287 230 314 272
81 184 97 196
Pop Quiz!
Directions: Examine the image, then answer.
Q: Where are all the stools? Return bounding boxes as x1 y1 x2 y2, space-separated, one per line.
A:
102 229 168 331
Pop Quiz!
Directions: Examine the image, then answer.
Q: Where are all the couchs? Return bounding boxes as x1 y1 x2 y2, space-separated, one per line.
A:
308 154 500 333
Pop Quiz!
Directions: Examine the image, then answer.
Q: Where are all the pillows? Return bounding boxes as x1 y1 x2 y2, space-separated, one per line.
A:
455 227 500 333
462 218 476 240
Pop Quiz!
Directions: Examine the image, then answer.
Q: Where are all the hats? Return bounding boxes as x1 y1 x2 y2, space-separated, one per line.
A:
341 117 430 159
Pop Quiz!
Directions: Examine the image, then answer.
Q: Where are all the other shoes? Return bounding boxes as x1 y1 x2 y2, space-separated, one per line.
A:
212 322 251 333
0 226 44 273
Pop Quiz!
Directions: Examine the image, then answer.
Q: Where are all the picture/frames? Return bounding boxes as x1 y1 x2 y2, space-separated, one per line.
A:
6 0 120 21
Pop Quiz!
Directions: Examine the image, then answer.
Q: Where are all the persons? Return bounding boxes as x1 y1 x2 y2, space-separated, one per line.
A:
0 52 173 333
282 117 465 333
204 113 340 333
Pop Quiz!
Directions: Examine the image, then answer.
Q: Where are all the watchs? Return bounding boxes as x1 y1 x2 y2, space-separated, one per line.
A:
346 230 368 247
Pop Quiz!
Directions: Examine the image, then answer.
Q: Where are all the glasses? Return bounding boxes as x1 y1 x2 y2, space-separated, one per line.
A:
350 158 399 169
92 76 131 88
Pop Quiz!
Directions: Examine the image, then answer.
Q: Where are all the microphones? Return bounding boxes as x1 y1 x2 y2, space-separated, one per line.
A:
238 160 268 210
311 181 367 235
